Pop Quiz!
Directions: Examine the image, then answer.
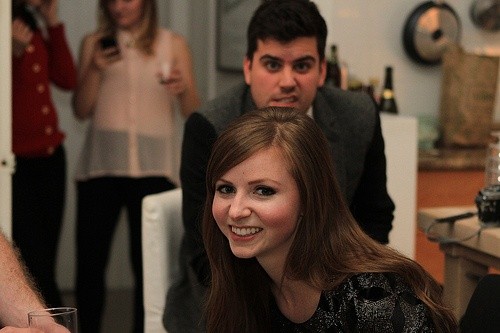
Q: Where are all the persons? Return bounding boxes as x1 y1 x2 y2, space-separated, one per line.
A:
71 1 196 333
11 0 79 323
202 107 457 332
0 229 69 333
163 0 395 333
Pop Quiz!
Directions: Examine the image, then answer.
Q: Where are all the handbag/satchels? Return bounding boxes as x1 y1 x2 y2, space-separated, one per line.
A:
474 184 500 224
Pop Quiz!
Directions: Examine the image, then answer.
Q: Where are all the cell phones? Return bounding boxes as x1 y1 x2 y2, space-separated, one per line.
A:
99 35 120 58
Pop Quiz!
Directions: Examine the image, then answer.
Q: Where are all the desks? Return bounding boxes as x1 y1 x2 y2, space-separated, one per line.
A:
417 206 500 319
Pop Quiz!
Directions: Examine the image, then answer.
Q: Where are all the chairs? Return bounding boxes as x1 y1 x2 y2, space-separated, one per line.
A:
379 113 419 261
143 186 183 331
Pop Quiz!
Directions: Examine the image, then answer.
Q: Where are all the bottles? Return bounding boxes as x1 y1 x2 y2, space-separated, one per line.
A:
378 66 398 116
327 44 341 85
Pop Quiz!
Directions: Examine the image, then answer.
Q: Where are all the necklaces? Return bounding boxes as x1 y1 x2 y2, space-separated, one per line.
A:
123 40 134 50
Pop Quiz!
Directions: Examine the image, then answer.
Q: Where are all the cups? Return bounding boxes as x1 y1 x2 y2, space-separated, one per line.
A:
155 55 177 88
27 307 78 333
